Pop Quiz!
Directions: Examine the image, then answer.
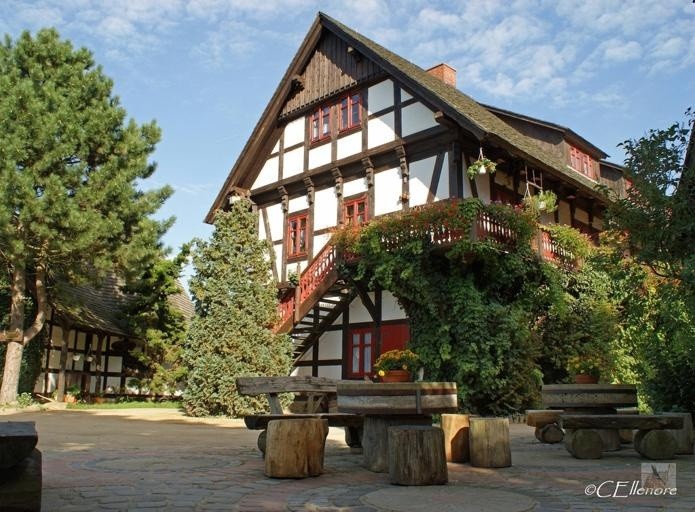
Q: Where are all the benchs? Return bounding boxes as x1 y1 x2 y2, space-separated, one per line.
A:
0 422 41 512
560 415 683 461
235 377 362 457
524 408 639 443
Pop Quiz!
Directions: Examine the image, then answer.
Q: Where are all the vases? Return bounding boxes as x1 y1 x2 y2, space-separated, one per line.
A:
380 370 410 382
575 374 597 384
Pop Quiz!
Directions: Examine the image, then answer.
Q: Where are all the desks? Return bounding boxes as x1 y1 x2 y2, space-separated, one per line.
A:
336 379 458 474
542 384 638 452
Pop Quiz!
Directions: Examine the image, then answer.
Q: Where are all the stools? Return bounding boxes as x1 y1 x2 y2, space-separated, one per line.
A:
389 426 449 487
442 414 481 463
663 411 695 454
268 419 328 479
469 417 512 467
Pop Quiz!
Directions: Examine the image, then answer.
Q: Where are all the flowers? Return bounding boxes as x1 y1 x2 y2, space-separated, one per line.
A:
566 351 609 378
373 348 419 377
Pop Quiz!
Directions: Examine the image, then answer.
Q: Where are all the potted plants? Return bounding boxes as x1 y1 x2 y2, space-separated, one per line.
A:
287 268 298 289
523 188 559 215
91 391 105 404
52 388 58 401
65 383 80 403
466 157 498 183
